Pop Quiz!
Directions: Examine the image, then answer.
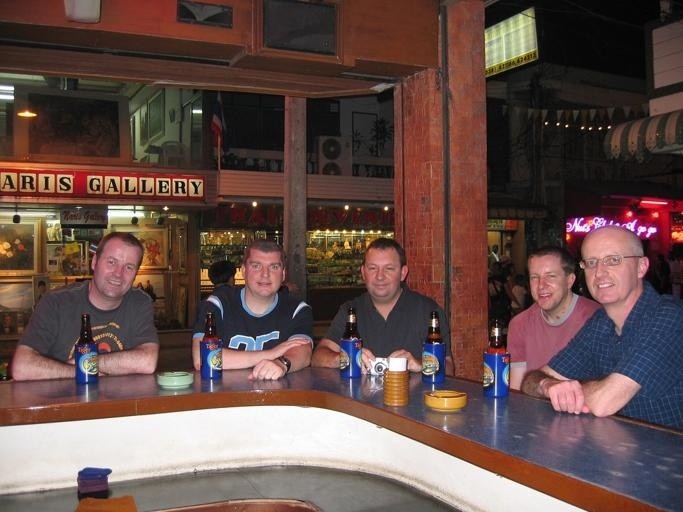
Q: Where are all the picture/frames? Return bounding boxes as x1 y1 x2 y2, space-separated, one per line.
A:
127 84 202 169
0 215 187 340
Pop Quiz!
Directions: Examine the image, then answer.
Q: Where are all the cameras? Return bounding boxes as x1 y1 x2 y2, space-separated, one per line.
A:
365 356 389 379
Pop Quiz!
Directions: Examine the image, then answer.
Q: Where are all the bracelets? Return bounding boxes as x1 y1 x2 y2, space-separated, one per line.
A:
276 356 291 375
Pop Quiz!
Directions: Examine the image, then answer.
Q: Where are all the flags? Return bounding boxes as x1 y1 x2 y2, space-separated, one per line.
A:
210 91 227 163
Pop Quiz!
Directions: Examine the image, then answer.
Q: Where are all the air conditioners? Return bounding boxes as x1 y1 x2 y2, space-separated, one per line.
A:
313 135 353 178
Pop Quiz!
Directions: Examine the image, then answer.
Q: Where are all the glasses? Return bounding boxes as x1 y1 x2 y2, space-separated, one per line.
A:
579 254 642 269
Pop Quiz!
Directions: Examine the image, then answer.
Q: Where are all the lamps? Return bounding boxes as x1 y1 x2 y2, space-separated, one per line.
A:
17 105 37 119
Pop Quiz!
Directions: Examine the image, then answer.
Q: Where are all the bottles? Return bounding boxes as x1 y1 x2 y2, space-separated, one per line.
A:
483 319 511 397
74 315 97 385
340 310 362 380
199 313 223 378
422 312 445 384
381 370 409 408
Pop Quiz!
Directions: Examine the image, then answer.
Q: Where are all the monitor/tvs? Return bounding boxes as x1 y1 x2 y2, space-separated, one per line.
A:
485 2 543 80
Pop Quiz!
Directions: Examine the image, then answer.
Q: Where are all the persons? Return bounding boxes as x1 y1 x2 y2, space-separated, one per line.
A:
488 245 528 345
652 254 683 296
520 225 683 432
11 231 161 382
506 245 603 391
310 236 455 377
192 238 314 381
208 260 237 288
35 280 49 304
